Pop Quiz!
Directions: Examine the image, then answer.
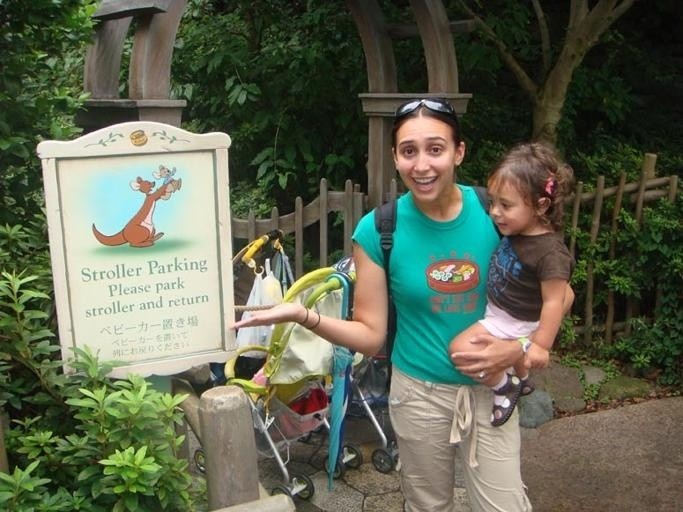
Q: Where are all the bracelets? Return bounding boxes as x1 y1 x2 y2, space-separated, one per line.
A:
298 307 309 326
307 311 321 331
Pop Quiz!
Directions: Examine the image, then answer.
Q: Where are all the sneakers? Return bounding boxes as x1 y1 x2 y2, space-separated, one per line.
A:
490 373 521 426
520 377 535 396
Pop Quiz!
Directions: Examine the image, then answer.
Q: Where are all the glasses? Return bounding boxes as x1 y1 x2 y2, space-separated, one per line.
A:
395 98 455 118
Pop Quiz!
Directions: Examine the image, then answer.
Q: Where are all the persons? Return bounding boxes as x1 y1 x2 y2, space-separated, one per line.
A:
449 142 579 428
228 96 575 512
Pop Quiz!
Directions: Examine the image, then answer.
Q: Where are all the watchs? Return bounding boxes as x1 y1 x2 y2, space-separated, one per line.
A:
517 336 533 352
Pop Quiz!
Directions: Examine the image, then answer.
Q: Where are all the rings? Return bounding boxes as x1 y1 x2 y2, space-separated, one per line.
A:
478 371 487 378
